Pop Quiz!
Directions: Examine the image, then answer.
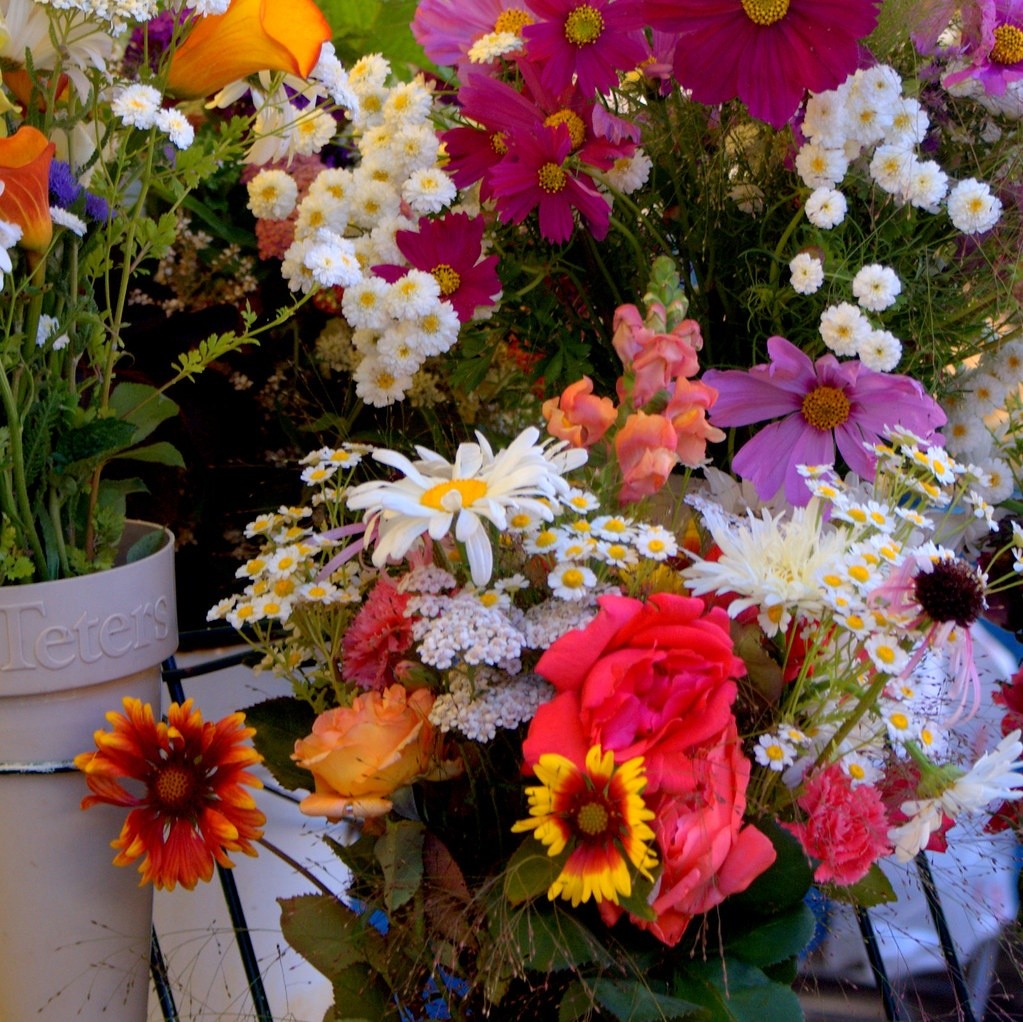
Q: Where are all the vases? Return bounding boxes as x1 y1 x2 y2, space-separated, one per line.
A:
0 521 177 1022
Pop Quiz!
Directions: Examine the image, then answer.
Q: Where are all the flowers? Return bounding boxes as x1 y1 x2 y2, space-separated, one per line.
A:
0 0 1023 1022
